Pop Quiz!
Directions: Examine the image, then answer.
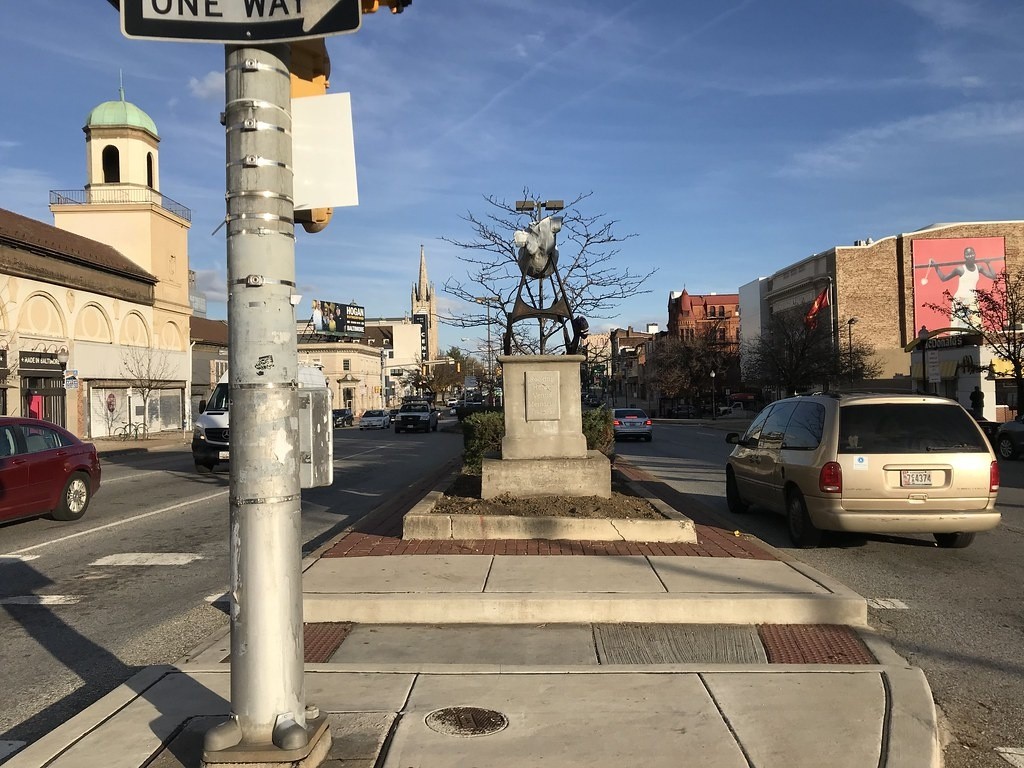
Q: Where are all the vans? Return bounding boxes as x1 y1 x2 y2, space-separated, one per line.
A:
728 393 766 410
192 366 327 476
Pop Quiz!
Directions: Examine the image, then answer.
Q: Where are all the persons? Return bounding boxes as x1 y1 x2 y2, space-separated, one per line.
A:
969 386 984 418
313 301 323 330
329 311 336 331
321 306 330 331
333 305 346 332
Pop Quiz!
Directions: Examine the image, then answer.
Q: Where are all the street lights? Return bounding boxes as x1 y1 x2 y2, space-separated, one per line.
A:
474 297 500 400
460 337 493 408
848 317 858 393
710 370 716 420
919 324 930 396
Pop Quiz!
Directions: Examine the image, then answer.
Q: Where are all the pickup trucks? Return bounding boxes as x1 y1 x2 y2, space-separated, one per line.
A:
718 401 756 417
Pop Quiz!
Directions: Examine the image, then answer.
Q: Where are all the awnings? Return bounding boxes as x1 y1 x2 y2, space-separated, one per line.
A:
0 383 18 388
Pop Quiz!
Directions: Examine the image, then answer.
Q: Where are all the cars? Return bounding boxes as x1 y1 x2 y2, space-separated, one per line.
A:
609 407 652 443
395 400 439 434
446 397 459 406
995 413 1024 461
582 394 600 407
334 409 354 428
671 405 697 419
449 406 461 417
359 409 391 430
388 408 399 423
0 415 102 528
725 393 1003 549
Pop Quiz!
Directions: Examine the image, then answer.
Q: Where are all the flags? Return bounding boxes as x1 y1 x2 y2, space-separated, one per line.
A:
809 284 830 316
